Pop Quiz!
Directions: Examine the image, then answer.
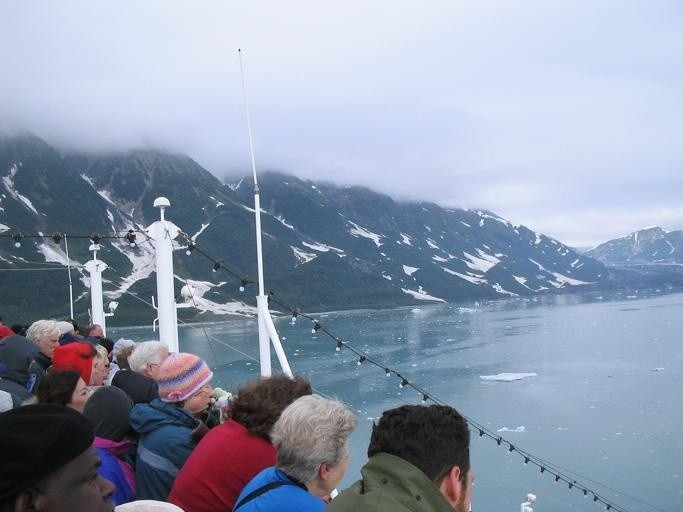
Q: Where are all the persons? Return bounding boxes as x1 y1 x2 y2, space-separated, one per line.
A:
1 319 475 512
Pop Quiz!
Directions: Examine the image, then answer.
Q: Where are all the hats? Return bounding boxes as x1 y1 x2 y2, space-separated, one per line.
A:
157 354 214 402
1 404 95 502
